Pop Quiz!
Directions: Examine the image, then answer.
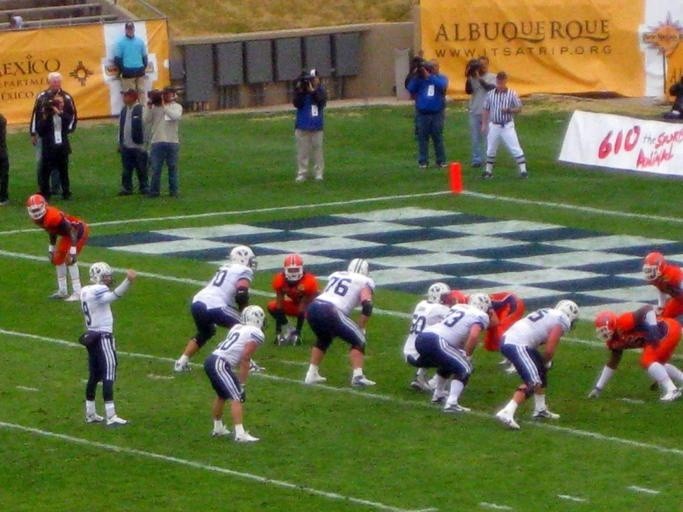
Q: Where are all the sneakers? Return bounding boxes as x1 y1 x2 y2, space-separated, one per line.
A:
50 291 68 298
211 428 232 436
411 359 560 428
85 412 104 423
234 433 260 443
106 415 128 425
65 294 80 302
659 390 682 403
173 332 377 387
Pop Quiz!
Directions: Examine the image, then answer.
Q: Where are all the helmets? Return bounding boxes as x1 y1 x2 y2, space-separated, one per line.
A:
427 282 580 323
27 194 48 221
643 251 664 282
88 260 112 284
226 246 370 328
596 311 616 342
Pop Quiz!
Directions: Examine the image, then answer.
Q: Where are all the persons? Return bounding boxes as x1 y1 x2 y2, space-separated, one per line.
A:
204 305 264 443
29 72 77 193
8 14 23 25
114 22 149 105
175 246 267 373
267 254 319 347
405 280 580 427
143 86 183 197
667 75 683 121
0 114 11 205
405 48 528 182
643 252 683 315
79 260 138 425
115 90 149 195
27 195 88 302
304 259 376 386
589 305 683 403
41 97 71 200
292 67 328 181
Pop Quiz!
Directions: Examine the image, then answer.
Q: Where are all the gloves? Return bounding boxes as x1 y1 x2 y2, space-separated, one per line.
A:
587 387 600 398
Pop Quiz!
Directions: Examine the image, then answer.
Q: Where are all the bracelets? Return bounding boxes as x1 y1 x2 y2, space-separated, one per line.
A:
69 245 77 255
47 244 54 254
646 311 656 327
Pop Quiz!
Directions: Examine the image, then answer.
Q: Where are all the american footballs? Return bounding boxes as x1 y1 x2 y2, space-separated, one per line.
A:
288 329 301 344
